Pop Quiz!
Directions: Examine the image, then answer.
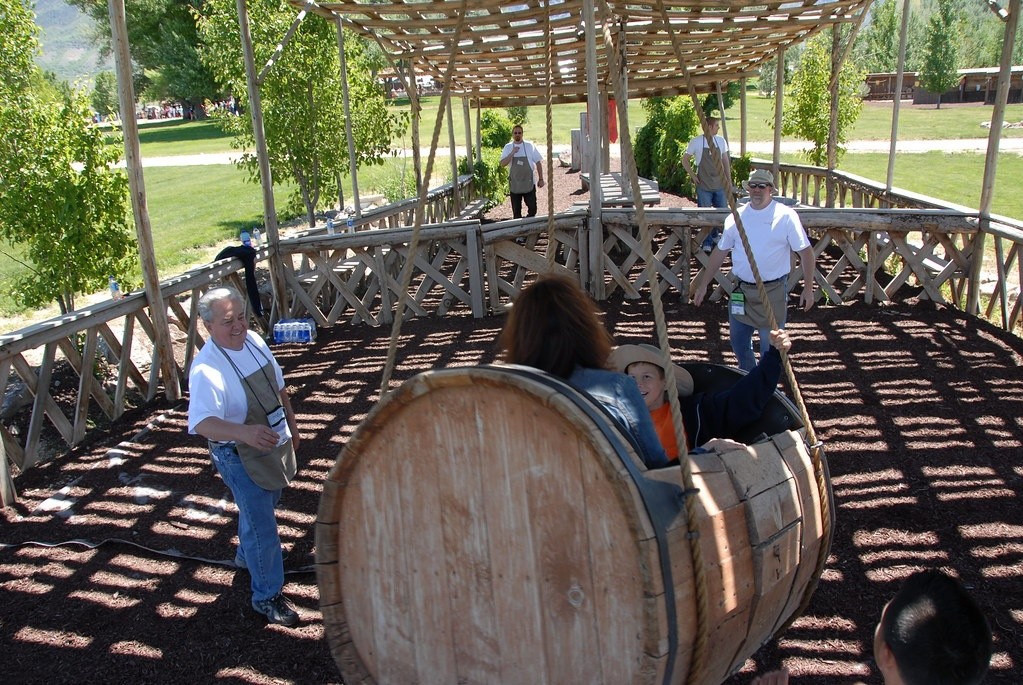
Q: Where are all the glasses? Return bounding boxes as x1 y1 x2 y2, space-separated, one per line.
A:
515 132 522 134
747 182 770 189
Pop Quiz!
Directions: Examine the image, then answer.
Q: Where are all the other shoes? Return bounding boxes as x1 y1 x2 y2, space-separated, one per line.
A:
703 245 712 252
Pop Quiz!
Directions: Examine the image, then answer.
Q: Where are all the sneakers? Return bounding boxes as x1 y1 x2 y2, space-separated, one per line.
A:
234 550 289 568
252 591 300 626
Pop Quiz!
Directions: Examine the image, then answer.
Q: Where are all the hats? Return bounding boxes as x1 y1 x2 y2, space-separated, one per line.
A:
742 170 778 195
604 343 694 402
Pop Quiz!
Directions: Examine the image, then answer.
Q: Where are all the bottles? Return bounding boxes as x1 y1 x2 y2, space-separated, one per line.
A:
327 217 335 235
347 215 355 234
272 318 316 343
253 226 263 245
241 229 251 247
109 275 122 302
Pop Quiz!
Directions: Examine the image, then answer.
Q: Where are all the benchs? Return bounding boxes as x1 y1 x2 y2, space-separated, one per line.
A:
564 199 590 260
731 184 814 208
295 195 489 287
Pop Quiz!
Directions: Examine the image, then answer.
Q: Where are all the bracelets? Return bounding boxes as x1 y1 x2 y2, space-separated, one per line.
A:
539 178 543 180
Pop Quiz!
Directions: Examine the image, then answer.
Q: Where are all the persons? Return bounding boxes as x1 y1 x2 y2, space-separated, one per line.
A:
186 285 303 625
692 167 815 372
496 272 671 472
93 95 239 123
681 117 731 254
605 330 791 463
871 567 992 685
498 125 545 219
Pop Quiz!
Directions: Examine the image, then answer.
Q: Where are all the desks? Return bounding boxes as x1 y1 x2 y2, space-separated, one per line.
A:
579 171 660 254
